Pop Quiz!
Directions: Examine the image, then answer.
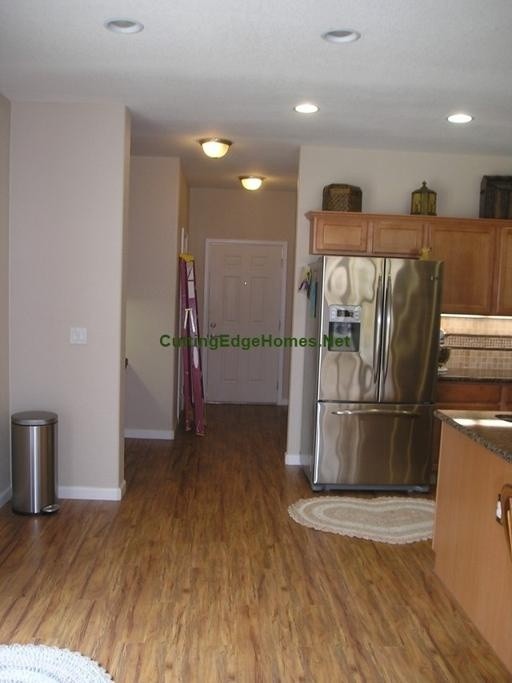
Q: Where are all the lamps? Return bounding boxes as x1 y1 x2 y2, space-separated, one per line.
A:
197 137 232 159
239 174 268 191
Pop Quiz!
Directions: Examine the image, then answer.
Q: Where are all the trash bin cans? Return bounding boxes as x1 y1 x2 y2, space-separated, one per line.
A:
11 411 60 516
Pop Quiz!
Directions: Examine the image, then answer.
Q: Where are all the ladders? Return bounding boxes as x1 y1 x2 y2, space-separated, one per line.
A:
178 254 207 437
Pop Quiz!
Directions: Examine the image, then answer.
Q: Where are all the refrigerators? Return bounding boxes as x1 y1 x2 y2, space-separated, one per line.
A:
300 256 442 492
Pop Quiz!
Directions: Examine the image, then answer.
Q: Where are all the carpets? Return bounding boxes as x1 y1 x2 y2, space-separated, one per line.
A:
287 494 436 546
0 643 116 683
